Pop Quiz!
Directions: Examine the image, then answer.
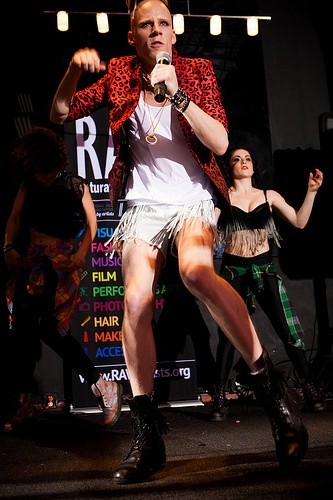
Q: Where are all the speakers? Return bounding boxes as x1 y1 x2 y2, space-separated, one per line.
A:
272 147 333 280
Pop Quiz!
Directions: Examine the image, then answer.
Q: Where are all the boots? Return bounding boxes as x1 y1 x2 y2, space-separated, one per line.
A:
249 367 309 470
90 374 123 428
112 391 171 484
300 377 327 414
209 380 232 423
2 393 35 433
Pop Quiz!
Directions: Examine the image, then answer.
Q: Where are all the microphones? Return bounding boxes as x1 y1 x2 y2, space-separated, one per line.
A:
154 51 172 103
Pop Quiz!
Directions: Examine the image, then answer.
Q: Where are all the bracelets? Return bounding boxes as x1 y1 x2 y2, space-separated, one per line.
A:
3 244 16 253
68 65 82 71
168 87 191 113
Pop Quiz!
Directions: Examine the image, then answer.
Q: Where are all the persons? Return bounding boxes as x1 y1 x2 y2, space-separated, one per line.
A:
202 146 331 421
50 0 309 485
4 127 123 432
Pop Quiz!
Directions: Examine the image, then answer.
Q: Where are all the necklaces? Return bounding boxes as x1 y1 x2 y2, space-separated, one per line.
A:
142 70 168 145
